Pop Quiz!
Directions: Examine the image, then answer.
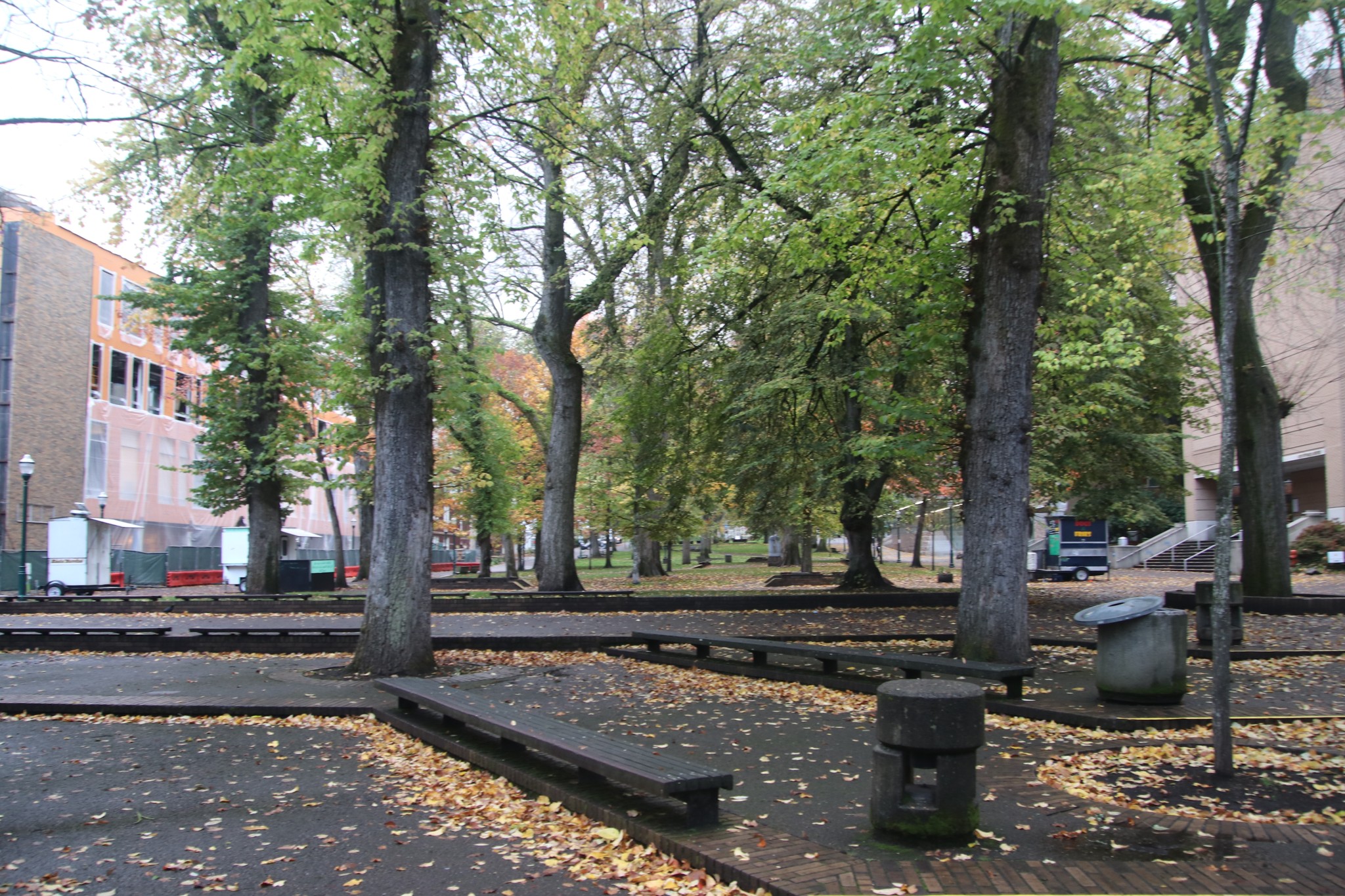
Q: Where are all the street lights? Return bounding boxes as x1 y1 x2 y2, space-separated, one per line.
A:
946 501 955 568
350 518 358 566
98 492 108 519
442 527 448 549
451 517 457 575
18 454 35 600
896 510 901 563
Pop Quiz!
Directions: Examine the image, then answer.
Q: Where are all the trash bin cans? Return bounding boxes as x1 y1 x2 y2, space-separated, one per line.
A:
1074 596 1190 704
725 555 732 562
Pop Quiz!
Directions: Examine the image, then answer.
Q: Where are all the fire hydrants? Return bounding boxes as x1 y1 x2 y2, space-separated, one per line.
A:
1289 550 1297 567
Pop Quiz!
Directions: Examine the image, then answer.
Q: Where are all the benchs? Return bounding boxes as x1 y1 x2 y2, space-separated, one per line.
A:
688 565 704 569
373 675 732 831
175 594 311 601
490 591 634 597
0 627 172 635
329 594 367 600
189 626 362 636
696 555 712 564
840 553 851 564
0 595 161 602
746 557 767 562
432 576 532 589
764 571 844 587
631 628 1033 695
431 593 469 599
830 547 840 553
